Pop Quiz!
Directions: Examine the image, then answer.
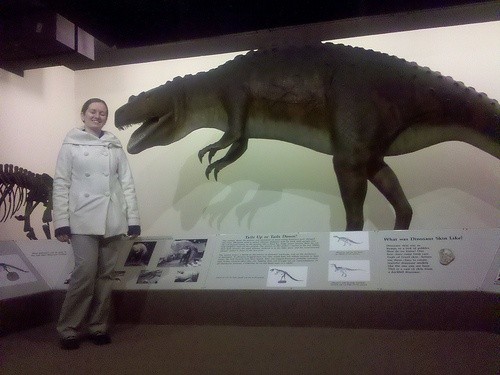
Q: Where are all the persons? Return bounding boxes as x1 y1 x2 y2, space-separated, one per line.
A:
51 98 141 351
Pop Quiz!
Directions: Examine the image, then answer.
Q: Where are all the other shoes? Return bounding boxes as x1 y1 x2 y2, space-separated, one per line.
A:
61 336 80 349
89 331 112 346
